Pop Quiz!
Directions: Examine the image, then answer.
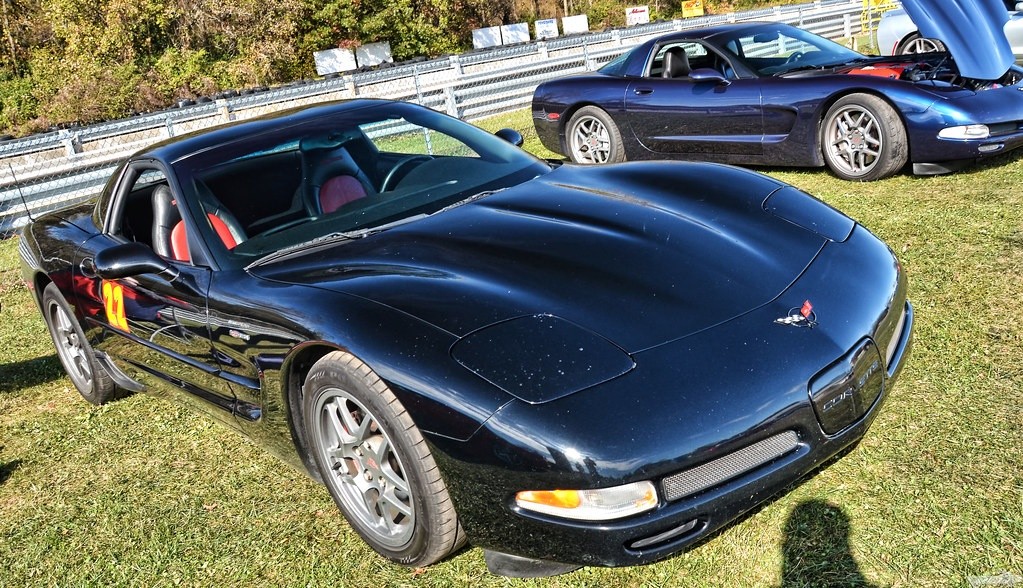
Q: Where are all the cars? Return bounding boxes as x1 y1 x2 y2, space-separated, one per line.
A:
876 2 1023 68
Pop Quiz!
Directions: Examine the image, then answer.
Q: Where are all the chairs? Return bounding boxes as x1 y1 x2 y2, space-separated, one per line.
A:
150 176 248 264
661 46 692 78
714 40 738 80
298 137 375 218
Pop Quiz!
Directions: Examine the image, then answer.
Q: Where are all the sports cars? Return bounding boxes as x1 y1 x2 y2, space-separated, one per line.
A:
18 98 915 577
532 0 1023 182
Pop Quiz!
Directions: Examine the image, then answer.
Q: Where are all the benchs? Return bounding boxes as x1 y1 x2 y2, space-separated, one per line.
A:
119 148 301 245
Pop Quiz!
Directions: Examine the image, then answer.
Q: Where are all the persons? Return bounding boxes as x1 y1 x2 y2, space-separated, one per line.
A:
653 16 665 24
